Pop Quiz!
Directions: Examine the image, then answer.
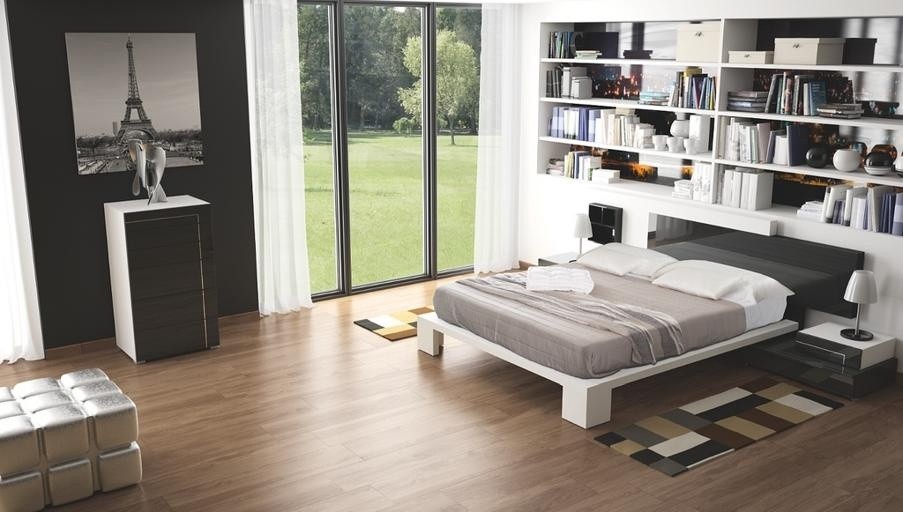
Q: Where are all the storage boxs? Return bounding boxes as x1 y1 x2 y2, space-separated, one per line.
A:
728 51 774 64
773 37 846 66
675 21 721 63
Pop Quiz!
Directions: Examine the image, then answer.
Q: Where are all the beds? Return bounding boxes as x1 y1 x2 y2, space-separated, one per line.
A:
416 212 865 430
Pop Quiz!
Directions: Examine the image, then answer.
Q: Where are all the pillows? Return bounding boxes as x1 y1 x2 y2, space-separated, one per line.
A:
576 241 796 305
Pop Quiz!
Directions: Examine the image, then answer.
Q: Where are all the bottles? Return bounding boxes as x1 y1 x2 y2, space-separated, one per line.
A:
804 140 903 178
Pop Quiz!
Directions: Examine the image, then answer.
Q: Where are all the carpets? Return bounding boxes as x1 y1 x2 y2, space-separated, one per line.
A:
593 375 845 477
353 306 434 341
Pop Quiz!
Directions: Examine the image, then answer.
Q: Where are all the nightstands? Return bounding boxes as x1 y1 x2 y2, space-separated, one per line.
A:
758 321 898 400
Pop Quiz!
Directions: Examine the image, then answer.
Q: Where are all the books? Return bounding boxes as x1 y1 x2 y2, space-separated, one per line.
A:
671 161 713 201
666 66 716 110
546 106 655 150
715 163 773 210
546 31 584 59
727 67 865 120
546 65 592 99
544 143 601 180
796 181 903 237
717 116 808 167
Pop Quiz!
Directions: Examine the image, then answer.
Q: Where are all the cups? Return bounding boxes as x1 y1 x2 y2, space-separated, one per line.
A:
666 135 685 154
651 134 668 151
682 137 701 158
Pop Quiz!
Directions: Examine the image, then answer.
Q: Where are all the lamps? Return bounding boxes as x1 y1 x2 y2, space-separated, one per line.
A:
840 269 878 341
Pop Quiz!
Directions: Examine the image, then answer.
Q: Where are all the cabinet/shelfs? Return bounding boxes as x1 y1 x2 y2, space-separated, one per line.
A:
518 3 903 375
103 194 220 365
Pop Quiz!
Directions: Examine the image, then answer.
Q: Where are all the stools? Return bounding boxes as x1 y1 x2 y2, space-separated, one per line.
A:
0 367 143 512
